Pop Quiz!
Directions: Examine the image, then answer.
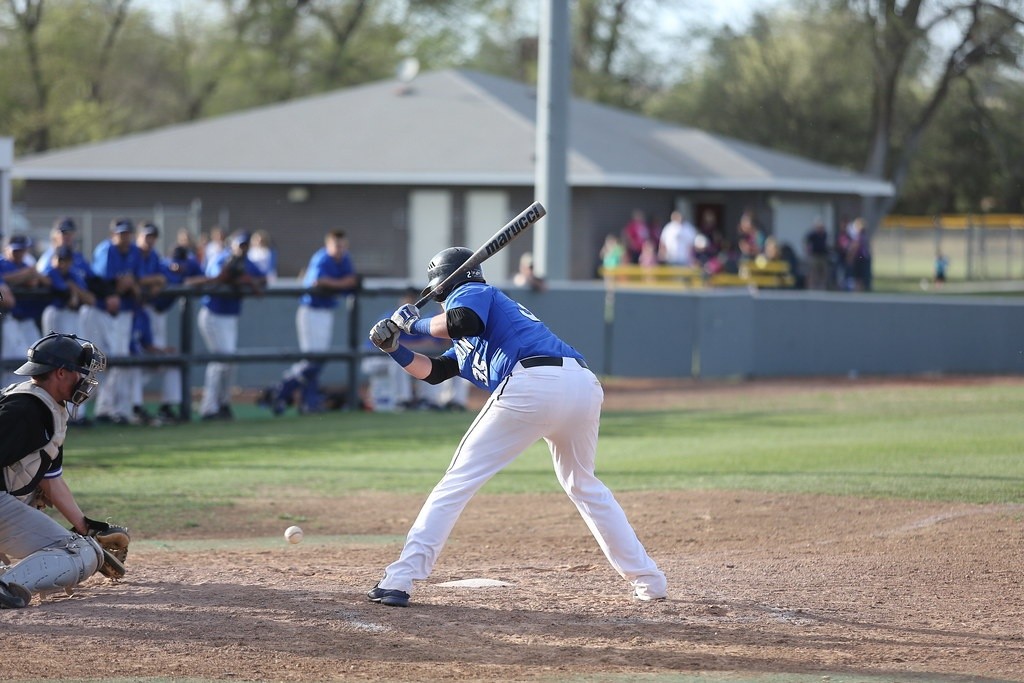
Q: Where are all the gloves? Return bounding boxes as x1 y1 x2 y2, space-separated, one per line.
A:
390 304 422 336
369 317 401 354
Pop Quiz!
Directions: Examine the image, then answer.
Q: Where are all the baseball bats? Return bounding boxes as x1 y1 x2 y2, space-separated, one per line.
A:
367 200 547 349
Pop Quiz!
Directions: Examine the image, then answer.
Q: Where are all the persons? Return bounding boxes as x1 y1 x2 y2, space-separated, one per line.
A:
0 330 106 609
600 206 802 294
0 213 276 425
514 251 547 289
256 226 363 415
363 247 668 606
805 217 872 291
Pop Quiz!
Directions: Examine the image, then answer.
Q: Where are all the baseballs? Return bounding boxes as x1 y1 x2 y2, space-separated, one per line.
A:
284 526 304 543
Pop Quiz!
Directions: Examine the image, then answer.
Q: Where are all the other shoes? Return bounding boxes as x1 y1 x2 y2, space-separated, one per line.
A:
368 582 410 606
632 589 667 603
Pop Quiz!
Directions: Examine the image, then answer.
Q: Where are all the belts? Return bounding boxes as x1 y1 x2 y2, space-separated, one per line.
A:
508 358 588 375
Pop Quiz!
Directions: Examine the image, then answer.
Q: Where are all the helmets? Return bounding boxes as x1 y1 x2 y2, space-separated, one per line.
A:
419 247 486 302
16 336 90 374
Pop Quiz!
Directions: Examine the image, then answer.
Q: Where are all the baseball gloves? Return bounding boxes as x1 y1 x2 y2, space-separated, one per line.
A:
92 523 131 579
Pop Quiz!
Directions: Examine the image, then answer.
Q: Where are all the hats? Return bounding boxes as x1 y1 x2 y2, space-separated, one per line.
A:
111 217 131 233
139 222 159 237
53 218 75 234
231 231 250 245
10 233 29 250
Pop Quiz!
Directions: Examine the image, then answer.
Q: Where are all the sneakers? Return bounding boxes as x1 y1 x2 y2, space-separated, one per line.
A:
0 582 23 609
